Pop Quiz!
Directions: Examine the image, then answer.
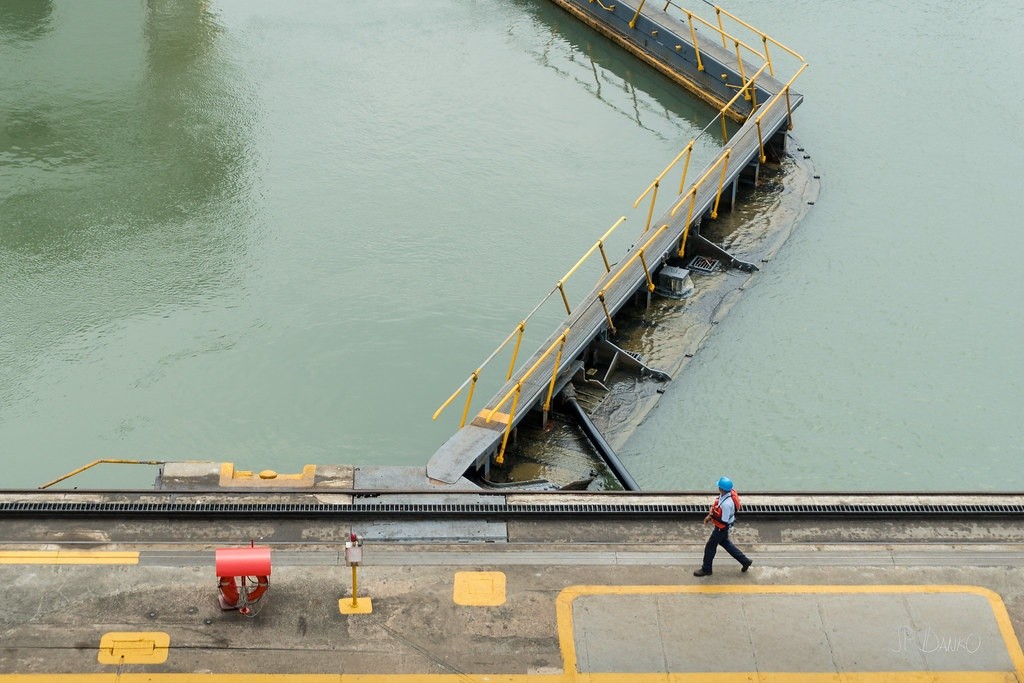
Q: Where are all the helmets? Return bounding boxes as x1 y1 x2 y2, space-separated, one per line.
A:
716 477 733 491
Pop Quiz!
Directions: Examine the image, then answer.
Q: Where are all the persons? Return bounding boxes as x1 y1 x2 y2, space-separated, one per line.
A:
693 476 753 577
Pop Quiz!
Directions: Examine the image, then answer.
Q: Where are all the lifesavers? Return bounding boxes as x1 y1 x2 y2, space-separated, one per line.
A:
219 575 268 601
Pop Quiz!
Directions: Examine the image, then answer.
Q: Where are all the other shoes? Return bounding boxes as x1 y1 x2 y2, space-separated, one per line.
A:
694 568 713 577
741 560 753 572
